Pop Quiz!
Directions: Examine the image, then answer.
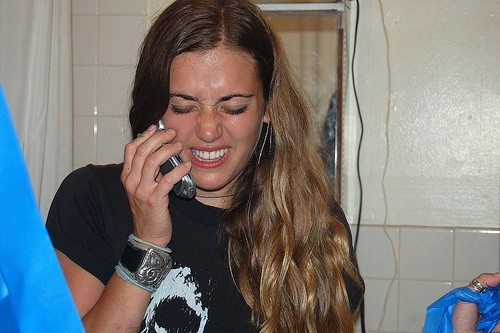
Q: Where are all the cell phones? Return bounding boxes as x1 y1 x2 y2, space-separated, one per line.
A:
139 119 195 199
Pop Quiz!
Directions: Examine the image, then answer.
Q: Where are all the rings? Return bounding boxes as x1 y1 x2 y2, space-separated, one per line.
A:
468 279 486 294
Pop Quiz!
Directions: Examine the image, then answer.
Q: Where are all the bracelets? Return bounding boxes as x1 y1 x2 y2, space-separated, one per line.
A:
115 234 172 293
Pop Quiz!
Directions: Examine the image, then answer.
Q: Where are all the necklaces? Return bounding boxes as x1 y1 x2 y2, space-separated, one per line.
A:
194 194 235 198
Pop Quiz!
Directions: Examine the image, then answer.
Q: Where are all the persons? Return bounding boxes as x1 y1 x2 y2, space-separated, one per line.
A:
44 0 366 333
451 272 500 333
